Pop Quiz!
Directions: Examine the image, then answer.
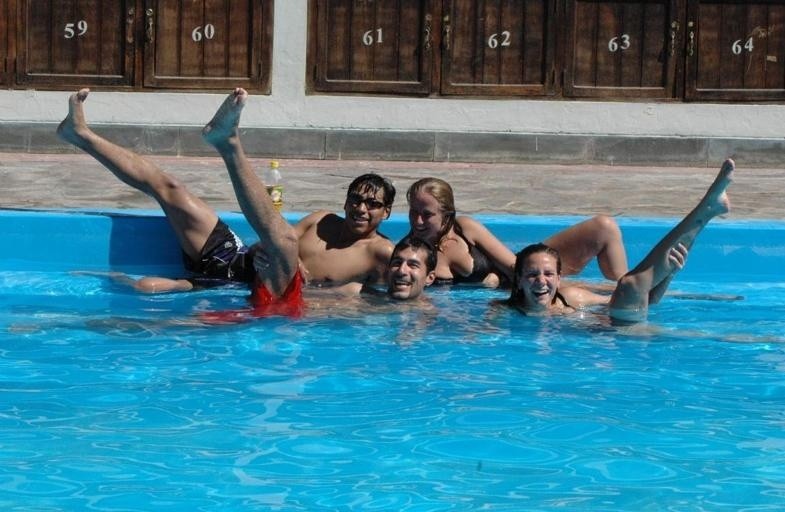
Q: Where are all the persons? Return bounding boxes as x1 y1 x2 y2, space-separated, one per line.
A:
480 156 785 345
198 85 439 322
389 175 747 304
56 89 399 304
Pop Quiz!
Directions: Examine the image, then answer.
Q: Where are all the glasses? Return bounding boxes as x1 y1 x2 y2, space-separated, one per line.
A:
347 192 386 208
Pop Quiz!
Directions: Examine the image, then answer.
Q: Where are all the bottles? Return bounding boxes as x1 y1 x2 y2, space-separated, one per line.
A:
264 160 284 212
